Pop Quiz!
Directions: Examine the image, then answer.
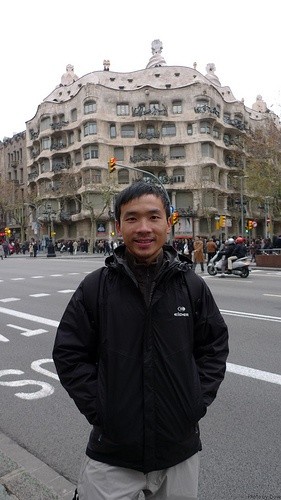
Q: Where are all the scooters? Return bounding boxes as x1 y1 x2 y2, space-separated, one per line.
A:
207 244 256 278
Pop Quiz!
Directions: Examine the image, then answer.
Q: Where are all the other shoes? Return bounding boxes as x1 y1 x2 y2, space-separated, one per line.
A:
219 274 225 278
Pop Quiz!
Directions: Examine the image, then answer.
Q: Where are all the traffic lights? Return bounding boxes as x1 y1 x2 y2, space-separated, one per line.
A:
220 215 227 228
248 220 254 231
173 212 179 225
109 158 116 173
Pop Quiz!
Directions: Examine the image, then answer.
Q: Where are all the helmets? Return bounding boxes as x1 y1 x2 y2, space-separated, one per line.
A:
227 238 234 245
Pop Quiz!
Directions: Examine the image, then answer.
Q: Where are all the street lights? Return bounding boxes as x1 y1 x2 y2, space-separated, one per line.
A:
233 176 249 238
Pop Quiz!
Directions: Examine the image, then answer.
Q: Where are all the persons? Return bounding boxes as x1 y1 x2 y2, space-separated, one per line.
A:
0 237 281 279
51 181 228 500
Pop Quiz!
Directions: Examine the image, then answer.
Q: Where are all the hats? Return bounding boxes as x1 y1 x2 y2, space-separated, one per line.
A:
236 237 243 243
